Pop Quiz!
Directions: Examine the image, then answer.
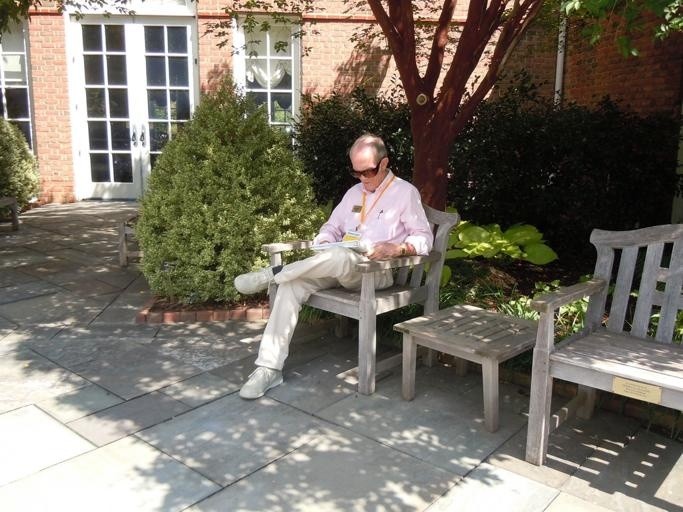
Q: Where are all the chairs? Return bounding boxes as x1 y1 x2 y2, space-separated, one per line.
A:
261 203 461 395
525 223 683 467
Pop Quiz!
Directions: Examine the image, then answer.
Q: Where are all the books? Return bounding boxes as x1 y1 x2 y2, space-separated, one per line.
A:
309 240 369 253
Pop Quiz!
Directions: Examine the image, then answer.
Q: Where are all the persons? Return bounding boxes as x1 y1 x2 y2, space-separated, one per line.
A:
235 133 435 401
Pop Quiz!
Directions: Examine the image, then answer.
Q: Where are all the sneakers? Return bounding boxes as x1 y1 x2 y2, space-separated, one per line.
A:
240 366 284 399
234 266 276 294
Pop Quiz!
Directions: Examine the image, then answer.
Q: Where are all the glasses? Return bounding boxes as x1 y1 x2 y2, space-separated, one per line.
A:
349 156 387 177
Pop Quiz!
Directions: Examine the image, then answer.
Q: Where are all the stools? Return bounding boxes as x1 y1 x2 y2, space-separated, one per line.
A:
118 213 144 267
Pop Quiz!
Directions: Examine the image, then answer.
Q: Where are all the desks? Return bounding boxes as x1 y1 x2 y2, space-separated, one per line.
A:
393 305 560 434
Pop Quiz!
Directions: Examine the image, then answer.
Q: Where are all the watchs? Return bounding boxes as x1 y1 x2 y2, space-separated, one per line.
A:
400 246 405 257
